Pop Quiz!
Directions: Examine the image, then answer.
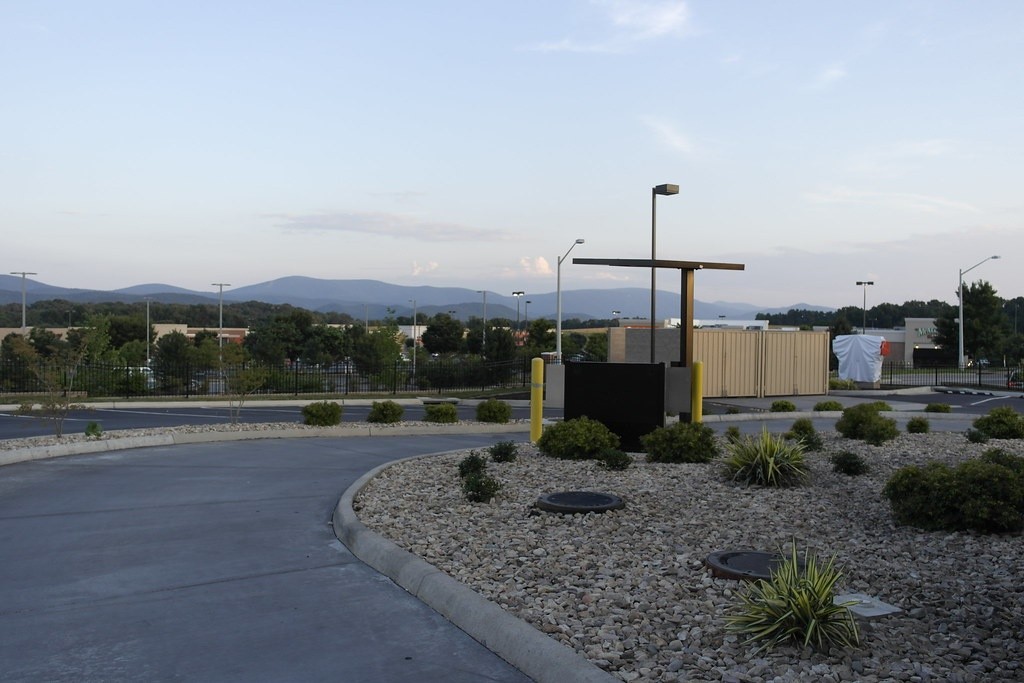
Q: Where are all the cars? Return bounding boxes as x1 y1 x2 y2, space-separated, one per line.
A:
976 359 989 367
1004 370 1024 389
114 367 153 375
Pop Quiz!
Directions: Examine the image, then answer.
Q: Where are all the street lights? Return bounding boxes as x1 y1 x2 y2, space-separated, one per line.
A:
66 310 76 328
649 184 679 363
556 239 584 365
144 297 153 367
409 299 417 377
10 272 37 336
449 311 455 319
212 283 230 394
526 300 531 349
959 255 1001 372
361 303 368 332
512 291 525 380
476 291 485 356
856 281 873 335
